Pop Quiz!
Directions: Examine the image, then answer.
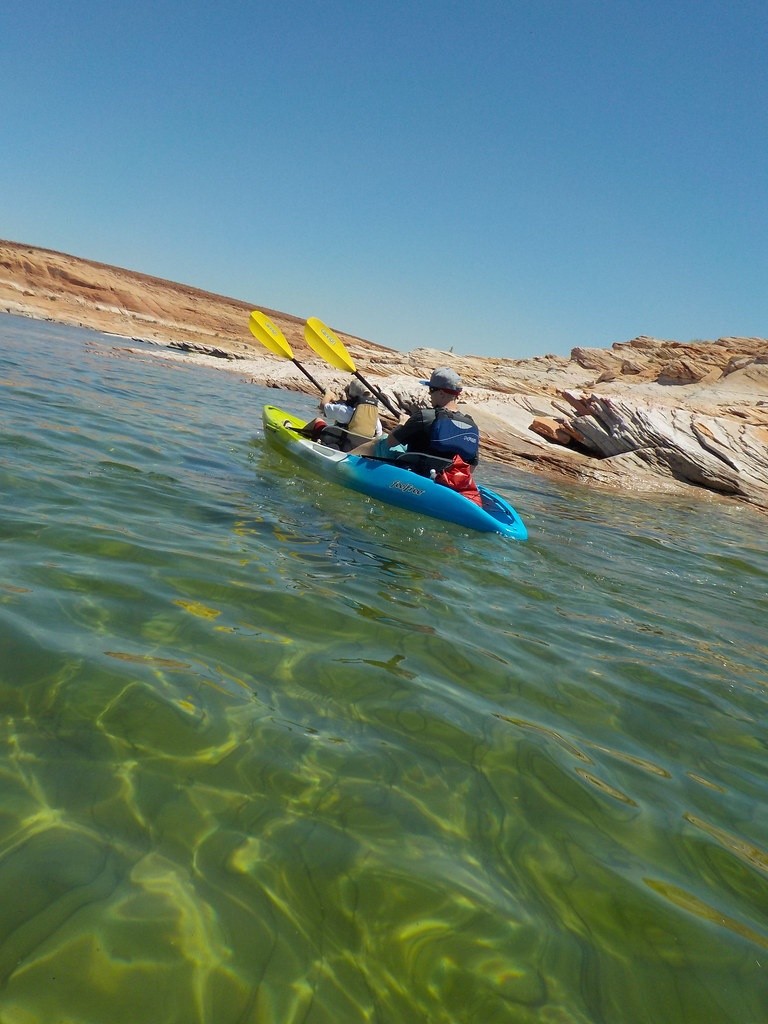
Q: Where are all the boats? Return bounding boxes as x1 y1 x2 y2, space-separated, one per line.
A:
262 404 530 542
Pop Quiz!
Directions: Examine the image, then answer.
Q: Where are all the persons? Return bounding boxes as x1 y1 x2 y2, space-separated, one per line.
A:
346 365 480 486
284 375 382 451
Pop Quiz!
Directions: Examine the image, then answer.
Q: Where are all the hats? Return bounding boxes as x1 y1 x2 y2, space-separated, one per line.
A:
349 378 373 398
419 367 463 391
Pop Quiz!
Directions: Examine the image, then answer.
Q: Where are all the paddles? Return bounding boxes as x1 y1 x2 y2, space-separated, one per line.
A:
304 317 401 419
247 311 325 393
